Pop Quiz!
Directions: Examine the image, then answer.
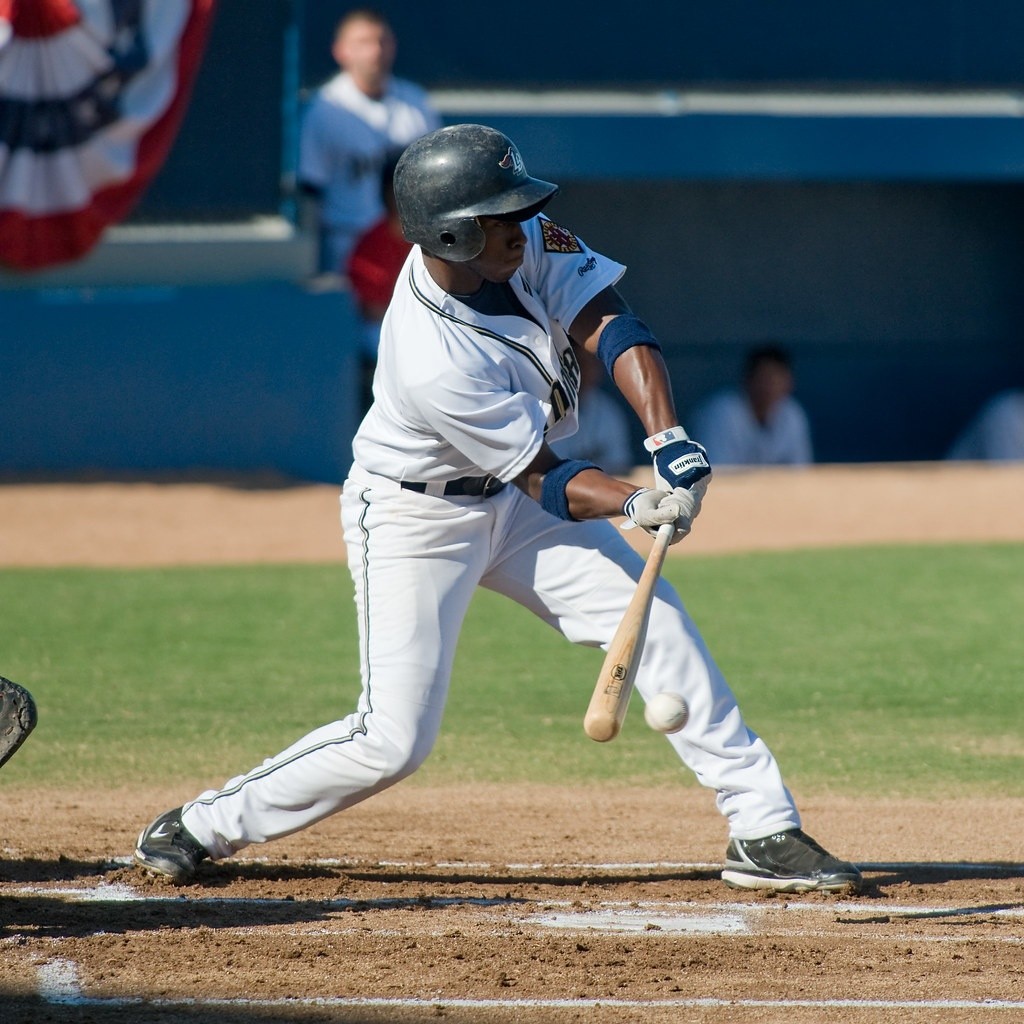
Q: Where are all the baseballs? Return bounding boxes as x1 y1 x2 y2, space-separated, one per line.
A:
643 692 689 735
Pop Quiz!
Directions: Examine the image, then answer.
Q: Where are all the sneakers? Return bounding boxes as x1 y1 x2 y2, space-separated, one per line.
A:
721 828 865 895
134 803 210 887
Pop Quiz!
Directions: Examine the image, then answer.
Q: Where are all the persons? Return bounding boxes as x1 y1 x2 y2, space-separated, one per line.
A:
544 322 1024 470
288 9 444 389
133 117 860 889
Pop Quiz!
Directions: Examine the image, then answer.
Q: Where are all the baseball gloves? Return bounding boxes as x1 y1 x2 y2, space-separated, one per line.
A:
0 677 38 766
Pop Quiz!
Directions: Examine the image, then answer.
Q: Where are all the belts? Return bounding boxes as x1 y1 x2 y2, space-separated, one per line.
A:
400 471 508 498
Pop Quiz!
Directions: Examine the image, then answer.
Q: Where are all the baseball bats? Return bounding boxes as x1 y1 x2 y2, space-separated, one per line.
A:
582 521 676 743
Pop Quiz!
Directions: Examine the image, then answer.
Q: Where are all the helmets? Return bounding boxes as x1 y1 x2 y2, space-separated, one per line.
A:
393 123 559 263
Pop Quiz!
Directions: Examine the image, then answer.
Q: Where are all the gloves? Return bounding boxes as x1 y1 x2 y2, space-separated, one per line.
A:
643 424 714 518
620 486 700 546
